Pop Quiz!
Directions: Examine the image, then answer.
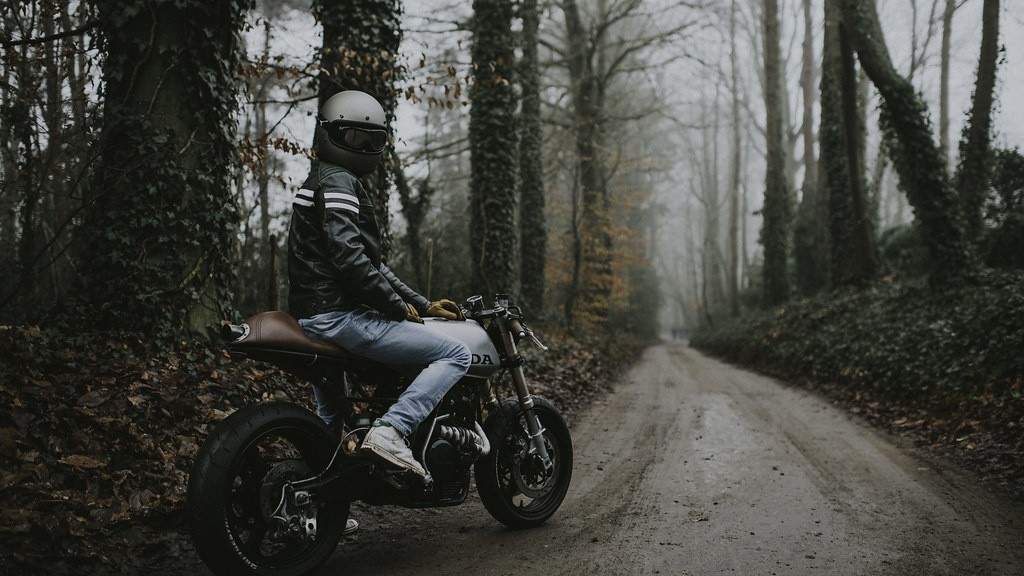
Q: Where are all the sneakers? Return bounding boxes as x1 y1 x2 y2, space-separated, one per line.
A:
360 418 427 479
303 516 358 539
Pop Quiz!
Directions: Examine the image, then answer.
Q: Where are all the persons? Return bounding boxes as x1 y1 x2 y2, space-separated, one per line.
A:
288 90 472 535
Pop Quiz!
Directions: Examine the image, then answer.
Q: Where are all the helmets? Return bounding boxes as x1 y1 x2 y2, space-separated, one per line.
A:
312 90 387 174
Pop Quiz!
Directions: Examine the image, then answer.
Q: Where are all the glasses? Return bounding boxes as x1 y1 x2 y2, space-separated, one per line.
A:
331 126 387 151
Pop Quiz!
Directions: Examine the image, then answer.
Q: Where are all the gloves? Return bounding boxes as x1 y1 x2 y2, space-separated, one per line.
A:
425 299 467 318
405 302 424 324
